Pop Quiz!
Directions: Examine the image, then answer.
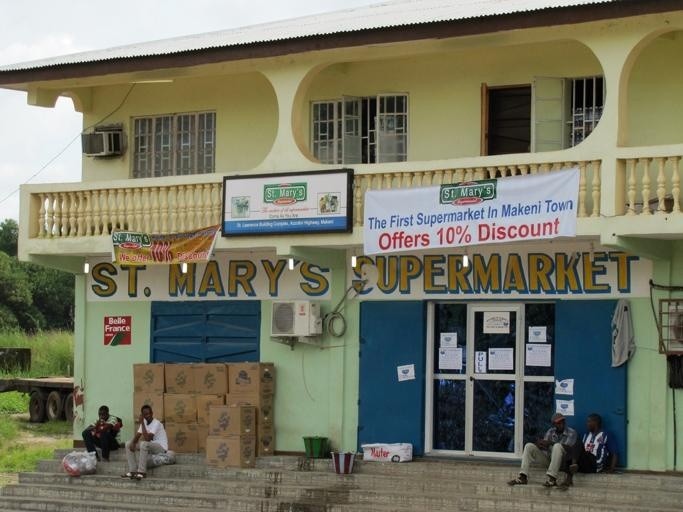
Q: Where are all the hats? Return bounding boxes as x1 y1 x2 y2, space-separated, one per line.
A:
551 413 566 424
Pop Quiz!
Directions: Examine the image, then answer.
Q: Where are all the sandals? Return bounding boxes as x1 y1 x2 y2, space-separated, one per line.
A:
120 471 147 480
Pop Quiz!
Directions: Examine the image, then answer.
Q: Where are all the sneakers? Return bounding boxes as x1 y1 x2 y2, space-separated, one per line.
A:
507 476 527 485
543 477 556 486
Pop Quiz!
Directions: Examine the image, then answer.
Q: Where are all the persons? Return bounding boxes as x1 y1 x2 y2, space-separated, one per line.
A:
119 405 168 479
505 413 579 487
82 405 124 461
556 414 612 487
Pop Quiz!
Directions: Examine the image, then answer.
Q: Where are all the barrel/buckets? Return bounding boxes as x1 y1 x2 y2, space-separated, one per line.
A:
330 450 355 475
302 435 329 459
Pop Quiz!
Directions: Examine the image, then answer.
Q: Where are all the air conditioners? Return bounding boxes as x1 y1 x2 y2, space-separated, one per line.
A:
78 128 122 158
267 299 325 342
664 304 682 354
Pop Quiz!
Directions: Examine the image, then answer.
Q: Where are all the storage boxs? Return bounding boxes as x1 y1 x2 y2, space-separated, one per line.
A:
130 361 276 468
360 441 412 465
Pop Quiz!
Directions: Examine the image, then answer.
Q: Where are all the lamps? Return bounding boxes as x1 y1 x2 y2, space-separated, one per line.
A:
84 257 90 274
587 240 595 264
182 263 187 274
462 246 470 268
351 249 357 268
287 256 295 271
